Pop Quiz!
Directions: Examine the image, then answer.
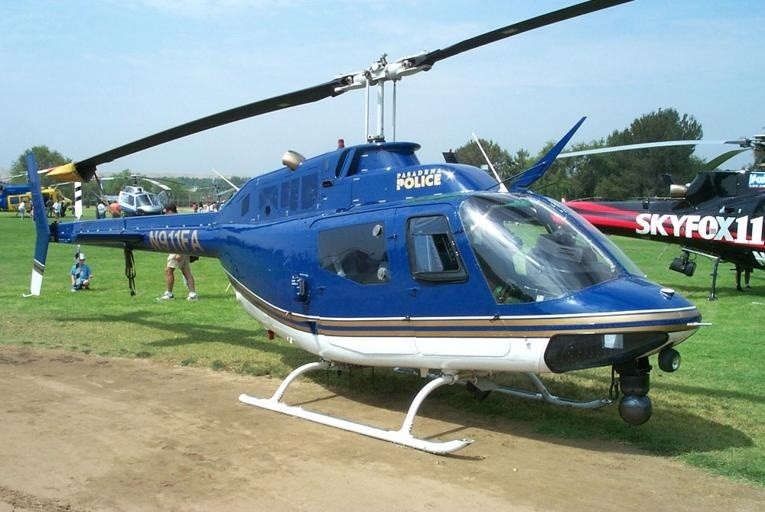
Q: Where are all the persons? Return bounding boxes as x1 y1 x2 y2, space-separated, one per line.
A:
735 263 753 291
45 197 53 217
160 204 198 300
18 200 25 220
109 200 122 218
191 199 224 215
95 200 101 218
96 201 105 218
61 196 66 216
52 199 61 219
70 253 93 291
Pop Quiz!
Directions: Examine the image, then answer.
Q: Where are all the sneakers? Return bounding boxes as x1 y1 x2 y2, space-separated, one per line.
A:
186 295 198 300
161 294 176 300
75 253 85 260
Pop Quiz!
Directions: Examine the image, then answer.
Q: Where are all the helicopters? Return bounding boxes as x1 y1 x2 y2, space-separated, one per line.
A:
23 0 711 460
